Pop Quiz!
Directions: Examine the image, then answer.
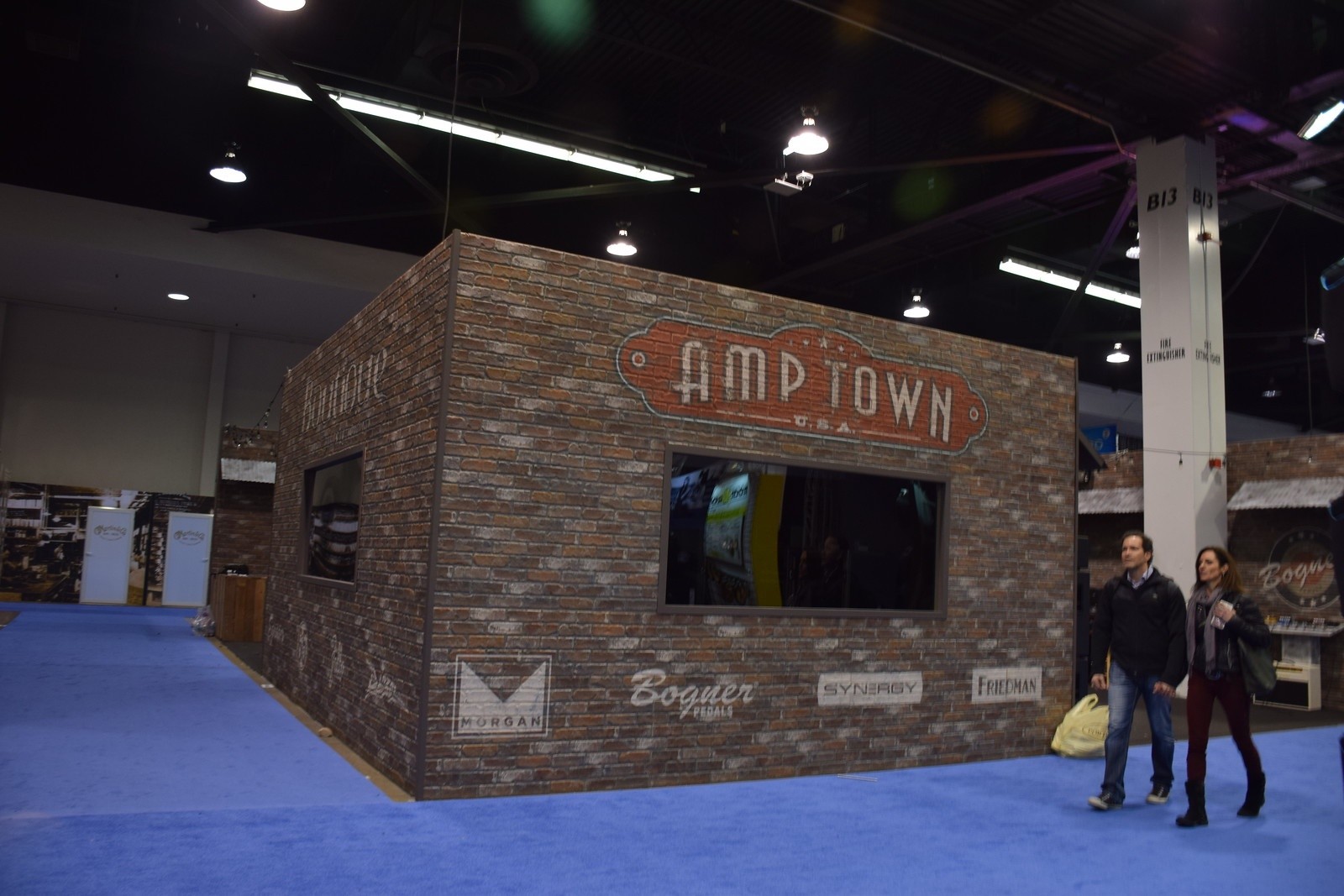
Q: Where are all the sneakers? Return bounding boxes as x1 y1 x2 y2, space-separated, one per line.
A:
1089 788 1124 810
1146 783 1170 803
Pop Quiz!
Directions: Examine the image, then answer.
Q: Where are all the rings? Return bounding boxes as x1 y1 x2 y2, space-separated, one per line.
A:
1091 683 1095 688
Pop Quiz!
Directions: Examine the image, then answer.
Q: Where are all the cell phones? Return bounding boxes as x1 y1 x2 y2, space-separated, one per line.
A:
1210 600 1233 630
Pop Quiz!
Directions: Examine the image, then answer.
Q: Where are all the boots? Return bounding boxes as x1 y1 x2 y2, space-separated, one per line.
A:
1236 773 1267 817
1176 783 1209 825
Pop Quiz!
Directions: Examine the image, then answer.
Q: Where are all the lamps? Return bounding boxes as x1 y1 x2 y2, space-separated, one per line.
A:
1105 342 1130 362
606 226 639 255
998 246 1141 312
1298 96 1344 138
788 108 830 155
903 287 931 319
207 131 251 182
1126 230 1140 258
248 66 702 182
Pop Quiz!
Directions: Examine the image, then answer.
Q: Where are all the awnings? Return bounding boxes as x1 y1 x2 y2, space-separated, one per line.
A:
1227 477 1344 510
1079 430 1109 472
1078 485 1144 513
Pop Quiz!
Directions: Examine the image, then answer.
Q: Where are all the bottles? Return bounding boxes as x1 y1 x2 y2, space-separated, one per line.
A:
1264 614 1326 630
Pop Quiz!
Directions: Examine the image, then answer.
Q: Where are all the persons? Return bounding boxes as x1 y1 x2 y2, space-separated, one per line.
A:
786 534 868 608
1155 547 1269 830
1086 531 1187 811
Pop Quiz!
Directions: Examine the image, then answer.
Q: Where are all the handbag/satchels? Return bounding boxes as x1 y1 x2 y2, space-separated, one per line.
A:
1234 635 1277 694
1048 694 1111 758
